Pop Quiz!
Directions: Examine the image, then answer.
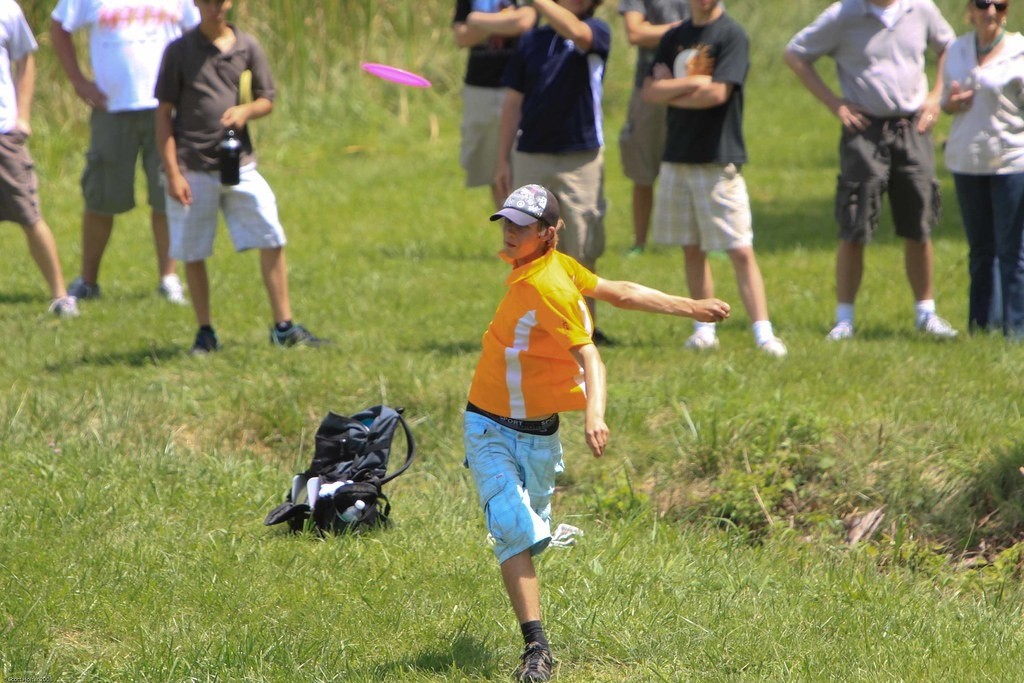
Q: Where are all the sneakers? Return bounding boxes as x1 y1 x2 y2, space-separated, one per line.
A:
48 295 80 318
754 334 788 357
516 642 553 683
687 330 720 352
65 278 101 300
620 246 647 259
157 272 188 305
825 320 854 340
270 323 337 347
189 329 217 355
914 312 959 338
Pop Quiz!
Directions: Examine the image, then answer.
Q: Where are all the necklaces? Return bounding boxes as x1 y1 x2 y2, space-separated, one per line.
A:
975 27 1005 55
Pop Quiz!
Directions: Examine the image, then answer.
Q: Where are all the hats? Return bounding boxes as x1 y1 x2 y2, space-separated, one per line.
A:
490 184 560 229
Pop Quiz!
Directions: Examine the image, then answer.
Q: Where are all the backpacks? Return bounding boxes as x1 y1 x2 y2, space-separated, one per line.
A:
263 406 414 537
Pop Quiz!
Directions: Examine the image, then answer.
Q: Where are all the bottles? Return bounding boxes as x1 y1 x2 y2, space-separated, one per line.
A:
340 500 366 524
217 123 242 184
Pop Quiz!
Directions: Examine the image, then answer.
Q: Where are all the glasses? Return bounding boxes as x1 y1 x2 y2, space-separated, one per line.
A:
974 0 1009 13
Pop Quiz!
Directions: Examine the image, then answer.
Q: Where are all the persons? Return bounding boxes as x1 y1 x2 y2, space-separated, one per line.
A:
153 1 332 360
51 0 201 305
1 0 77 319
639 1 788 360
459 184 733 682
449 0 540 214
939 0 1024 352
491 0 620 345
781 0 962 345
618 1 728 261
